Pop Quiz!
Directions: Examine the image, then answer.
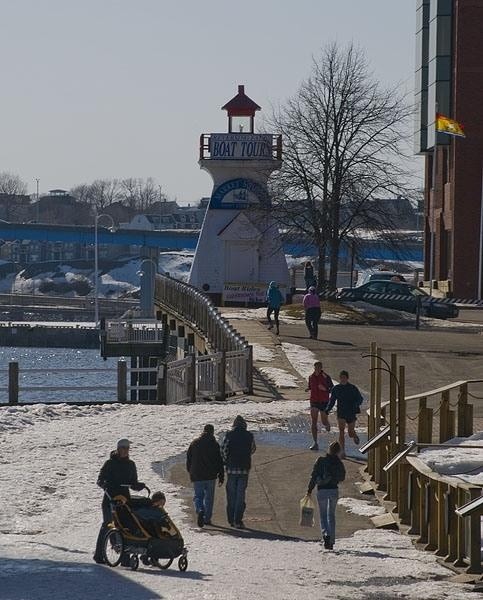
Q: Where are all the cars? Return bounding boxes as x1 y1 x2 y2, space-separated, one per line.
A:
357 271 409 294
338 280 457 318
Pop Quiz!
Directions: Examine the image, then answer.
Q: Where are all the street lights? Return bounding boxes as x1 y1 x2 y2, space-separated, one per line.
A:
93 214 115 323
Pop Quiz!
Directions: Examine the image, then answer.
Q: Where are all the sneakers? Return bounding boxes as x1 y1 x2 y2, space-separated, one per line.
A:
310 444 319 450
354 435 359 444
235 524 245 529
198 510 204 527
324 536 330 548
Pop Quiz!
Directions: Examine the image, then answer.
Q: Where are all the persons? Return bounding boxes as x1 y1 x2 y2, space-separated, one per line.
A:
303 260 315 291
304 363 334 450
137 491 168 537
91 439 141 564
185 423 224 527
324 369 363 460
265 280 282 336
222 414 256 529
302 286 322 340
305 440 346 549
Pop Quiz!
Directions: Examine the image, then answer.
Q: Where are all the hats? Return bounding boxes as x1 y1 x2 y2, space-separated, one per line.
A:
117 439 130 451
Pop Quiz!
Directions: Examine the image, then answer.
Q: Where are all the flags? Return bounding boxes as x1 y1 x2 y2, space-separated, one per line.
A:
434 112 465 138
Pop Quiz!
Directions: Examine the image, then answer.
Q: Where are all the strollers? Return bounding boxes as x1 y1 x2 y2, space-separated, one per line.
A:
100 483 186 572
